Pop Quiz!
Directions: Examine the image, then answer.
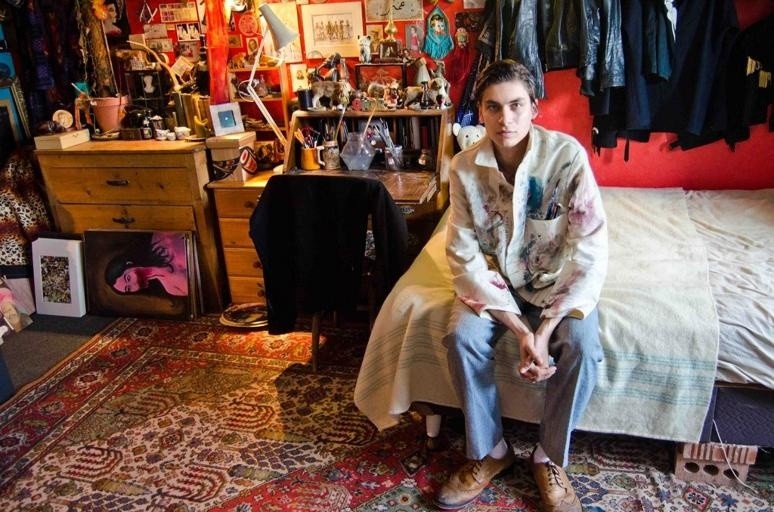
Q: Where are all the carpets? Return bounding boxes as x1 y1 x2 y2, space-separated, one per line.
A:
0 319 773 511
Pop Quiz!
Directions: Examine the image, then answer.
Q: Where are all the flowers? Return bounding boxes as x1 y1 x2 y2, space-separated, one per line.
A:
82 0 113 96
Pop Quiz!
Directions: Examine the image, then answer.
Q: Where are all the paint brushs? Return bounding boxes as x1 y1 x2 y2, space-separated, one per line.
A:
362 104 377 137
375 118 405 169
334 106 345 141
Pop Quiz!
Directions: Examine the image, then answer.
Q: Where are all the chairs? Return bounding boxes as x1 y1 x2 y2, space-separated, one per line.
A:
249 175 410 371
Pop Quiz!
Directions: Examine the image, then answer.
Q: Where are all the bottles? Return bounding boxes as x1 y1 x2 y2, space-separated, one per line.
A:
421 81 429 109
417 149 434 174
141 111 153 139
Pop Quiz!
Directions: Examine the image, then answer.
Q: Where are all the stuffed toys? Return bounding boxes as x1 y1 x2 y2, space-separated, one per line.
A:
357 34 372 64
452 122 486 151
310 78 453 110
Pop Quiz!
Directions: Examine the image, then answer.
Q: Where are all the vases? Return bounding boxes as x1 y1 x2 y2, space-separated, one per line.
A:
88 95 129 133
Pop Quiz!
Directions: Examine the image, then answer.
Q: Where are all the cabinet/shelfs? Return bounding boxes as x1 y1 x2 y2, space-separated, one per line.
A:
34 140 222 312
205 59 454 306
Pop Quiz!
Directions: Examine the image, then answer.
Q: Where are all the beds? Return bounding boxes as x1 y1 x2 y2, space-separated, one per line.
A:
353 188 773 446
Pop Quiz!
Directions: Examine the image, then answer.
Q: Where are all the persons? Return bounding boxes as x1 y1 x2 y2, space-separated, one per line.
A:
366 125 372 139
256 144 273 169
103 232 188 296
314 19 352 41
247 38 257 52
188 23 200 40
427 60 610 512
409 26 419 52
176 24 187 41
372 125 382 138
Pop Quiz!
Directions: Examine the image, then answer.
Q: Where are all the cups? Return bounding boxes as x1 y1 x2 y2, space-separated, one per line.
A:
301 147 321 170
317 141 341 170
155 129 170 141
297 90 312 110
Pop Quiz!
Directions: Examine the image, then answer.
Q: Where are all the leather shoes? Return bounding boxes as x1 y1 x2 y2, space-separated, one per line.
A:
432 440 516 509
529 441 583 512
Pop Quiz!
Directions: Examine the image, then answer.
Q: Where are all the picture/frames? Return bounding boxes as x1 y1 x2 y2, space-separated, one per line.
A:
82 231 196 320
208 100 246 137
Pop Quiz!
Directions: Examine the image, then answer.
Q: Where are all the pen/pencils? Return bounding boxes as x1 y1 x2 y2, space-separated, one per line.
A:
548 202 561 220
319 118 349 145
304 130 320 148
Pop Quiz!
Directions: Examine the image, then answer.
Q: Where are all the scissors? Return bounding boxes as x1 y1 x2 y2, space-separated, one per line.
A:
294 128 312 145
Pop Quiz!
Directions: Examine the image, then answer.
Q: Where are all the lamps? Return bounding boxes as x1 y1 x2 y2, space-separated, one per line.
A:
247 5 301 176
